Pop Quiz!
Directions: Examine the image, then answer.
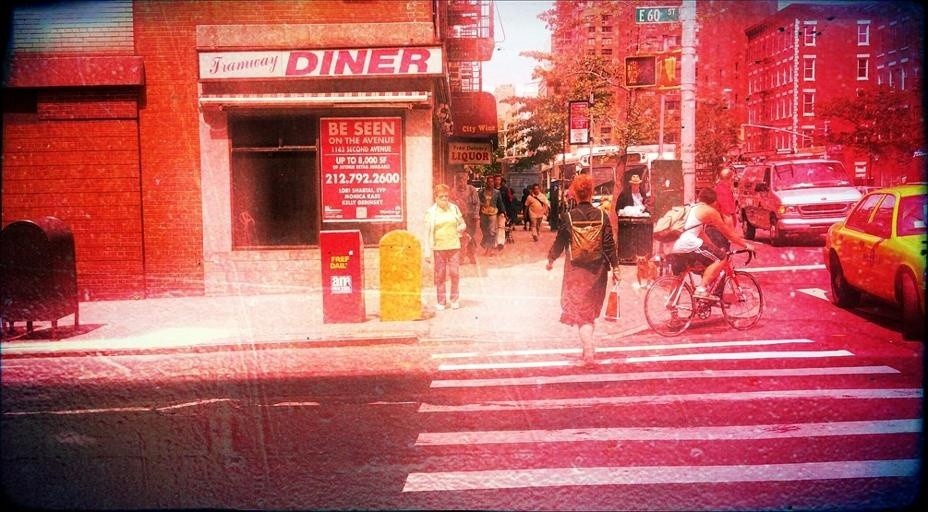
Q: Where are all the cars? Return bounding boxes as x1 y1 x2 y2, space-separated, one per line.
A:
822 183 928 320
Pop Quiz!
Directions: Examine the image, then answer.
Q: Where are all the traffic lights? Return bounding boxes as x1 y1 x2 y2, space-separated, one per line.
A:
623 55 659 89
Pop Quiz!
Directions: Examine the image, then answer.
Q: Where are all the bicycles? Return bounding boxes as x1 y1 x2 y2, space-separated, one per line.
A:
643 248 764 338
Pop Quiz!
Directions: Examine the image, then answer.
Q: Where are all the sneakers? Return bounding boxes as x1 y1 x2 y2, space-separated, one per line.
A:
459 257 465 265
436 304 445 311
470 256 477 264
489 250 495 256
480 249 485 254
692 285 718 301
450 303 459 309
584 361 597 370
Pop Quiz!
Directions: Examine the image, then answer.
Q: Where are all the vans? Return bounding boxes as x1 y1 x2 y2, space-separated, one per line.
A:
737 159 865 247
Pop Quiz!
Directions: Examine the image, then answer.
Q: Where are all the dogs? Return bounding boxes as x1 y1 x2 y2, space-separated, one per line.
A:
634 251 659 290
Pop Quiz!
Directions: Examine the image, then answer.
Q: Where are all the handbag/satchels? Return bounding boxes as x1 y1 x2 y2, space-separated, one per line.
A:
542 203 550 216
603 289 619 323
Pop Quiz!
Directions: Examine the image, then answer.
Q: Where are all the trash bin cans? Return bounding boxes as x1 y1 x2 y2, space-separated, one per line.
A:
0 216 81 339
618 215 653 265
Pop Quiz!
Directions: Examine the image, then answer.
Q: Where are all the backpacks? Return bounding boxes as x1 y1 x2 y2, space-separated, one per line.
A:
652 202 706 241
568 208 604 264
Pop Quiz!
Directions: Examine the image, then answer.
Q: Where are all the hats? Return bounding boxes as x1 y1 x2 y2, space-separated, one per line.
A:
629 175 642 185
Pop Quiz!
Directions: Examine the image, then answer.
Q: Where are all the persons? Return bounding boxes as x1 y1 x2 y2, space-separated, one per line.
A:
663 186 759 329
422 172 551 311
615 174 651 218
546 175 624 370
